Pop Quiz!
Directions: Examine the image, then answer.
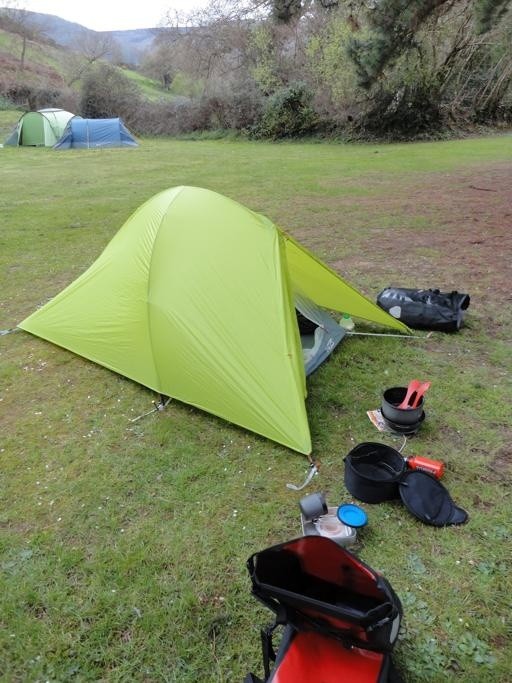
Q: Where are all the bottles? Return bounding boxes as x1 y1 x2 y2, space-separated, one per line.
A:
403 454 445 478
339 312 354 336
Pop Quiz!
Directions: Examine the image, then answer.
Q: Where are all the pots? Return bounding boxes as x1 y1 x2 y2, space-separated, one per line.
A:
381 386 425 423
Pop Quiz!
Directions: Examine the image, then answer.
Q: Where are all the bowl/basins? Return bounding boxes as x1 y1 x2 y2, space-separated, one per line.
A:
315 514 352 537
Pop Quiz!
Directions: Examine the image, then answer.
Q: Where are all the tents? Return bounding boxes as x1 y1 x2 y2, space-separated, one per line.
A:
52 115 140 148
0 107 75 147
16 184 414 457
